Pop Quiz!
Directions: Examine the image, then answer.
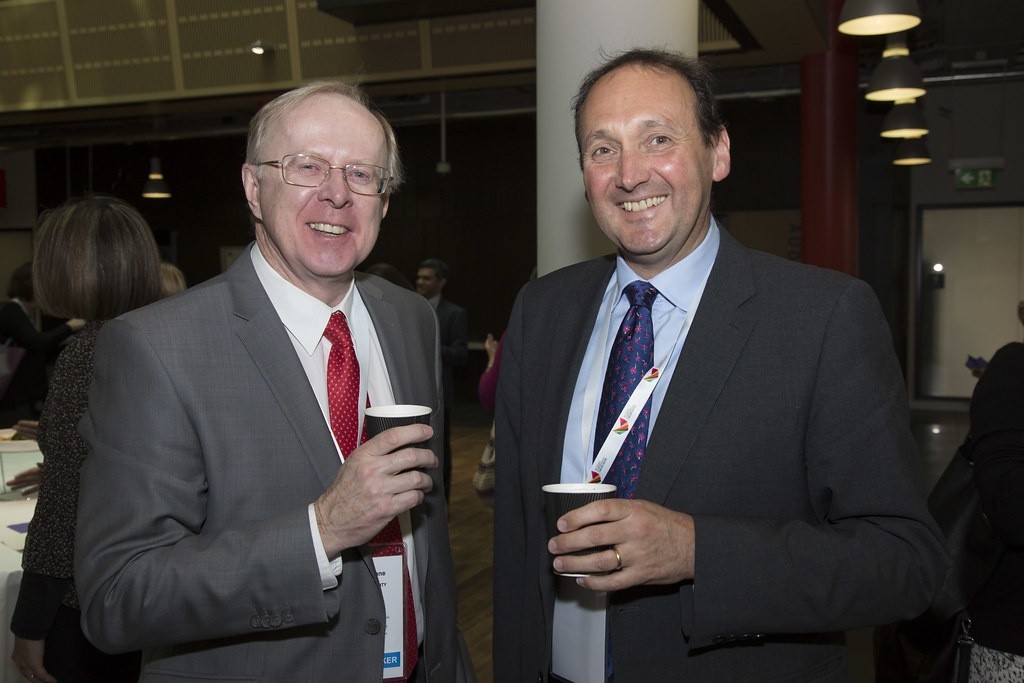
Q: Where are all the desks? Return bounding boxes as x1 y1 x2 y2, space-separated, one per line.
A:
0 421 55 683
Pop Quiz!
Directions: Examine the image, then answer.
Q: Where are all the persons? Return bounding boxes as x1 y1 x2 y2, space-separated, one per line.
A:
957 301 1024 683
368 259 508 411
71 81 457 683
491 50 954 683
1 194 187 683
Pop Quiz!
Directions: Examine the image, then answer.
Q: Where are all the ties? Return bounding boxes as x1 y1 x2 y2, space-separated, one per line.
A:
323 313 423 680
591 280 659 498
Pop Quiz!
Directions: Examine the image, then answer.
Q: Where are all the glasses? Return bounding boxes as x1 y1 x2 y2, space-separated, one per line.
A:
255 154 395 196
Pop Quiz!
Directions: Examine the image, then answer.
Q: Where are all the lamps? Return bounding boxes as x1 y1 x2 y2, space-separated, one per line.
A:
248 39 271 56
880 99 930 138
142 177 172 198
149 157 163 180
864 31 927 101
892 136 932 166
837 0 922 36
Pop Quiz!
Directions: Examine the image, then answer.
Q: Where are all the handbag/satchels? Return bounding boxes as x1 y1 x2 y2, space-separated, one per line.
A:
874 598 973 683
0 338 25 398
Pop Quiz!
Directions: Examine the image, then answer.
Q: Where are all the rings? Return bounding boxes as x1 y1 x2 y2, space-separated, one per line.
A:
614 548 622 570
27 674 36 680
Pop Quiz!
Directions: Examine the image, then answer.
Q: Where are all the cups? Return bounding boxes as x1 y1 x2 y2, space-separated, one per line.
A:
363 405 432 476
543 483 617 577
